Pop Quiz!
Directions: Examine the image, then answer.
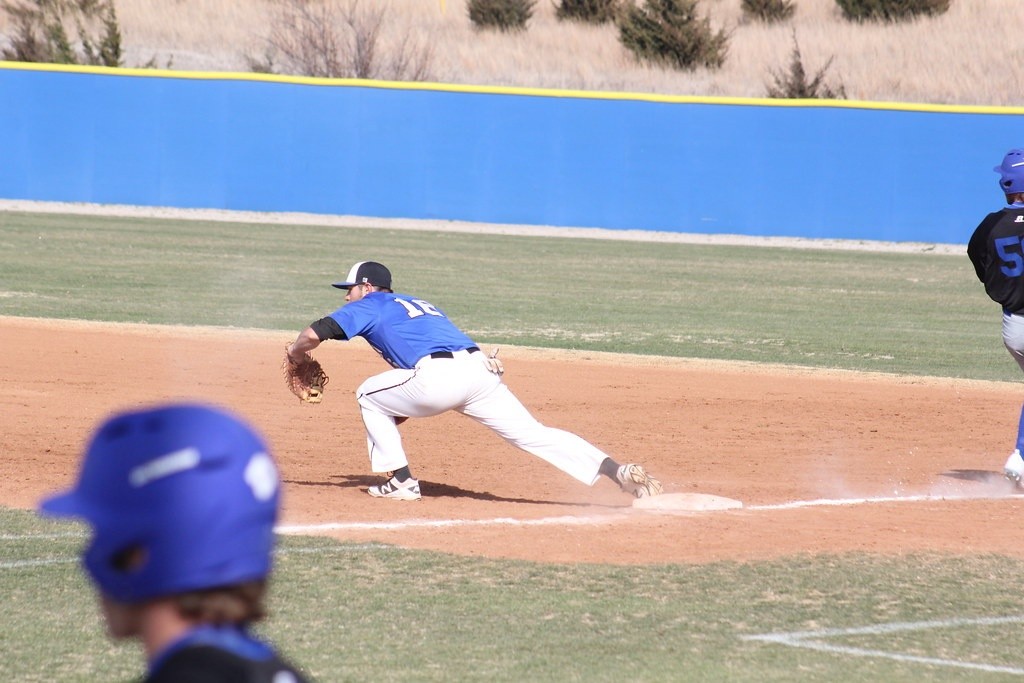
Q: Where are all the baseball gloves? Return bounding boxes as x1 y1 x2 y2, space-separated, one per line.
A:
281 338 330 405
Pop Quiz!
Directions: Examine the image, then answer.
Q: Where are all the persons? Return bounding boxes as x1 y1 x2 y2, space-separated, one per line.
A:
36 402 309 683
966 149 1024 492
281 262 665 501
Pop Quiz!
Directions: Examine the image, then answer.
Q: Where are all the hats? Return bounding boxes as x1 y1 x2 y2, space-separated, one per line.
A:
331 261 394 293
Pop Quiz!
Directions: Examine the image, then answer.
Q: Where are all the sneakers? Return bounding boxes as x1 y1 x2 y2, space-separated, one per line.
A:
616 464 664 499
1004 449 1024 490
368 471 421 500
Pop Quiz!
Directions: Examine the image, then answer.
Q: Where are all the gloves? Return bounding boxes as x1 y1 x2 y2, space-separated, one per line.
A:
483 347 504 376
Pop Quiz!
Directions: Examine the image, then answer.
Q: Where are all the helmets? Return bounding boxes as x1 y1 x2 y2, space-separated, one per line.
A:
38 404 281 603
994 149 1024 194
